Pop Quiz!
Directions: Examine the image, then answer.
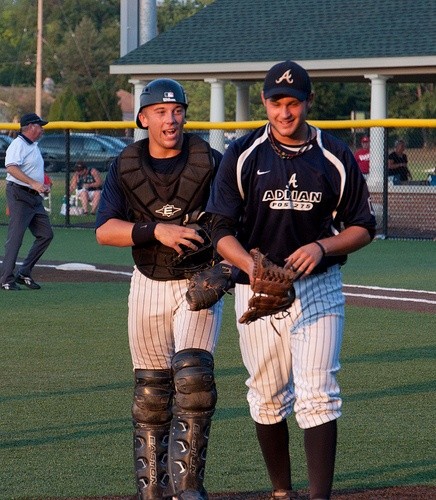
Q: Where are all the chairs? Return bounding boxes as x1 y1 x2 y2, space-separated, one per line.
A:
43 192 52 215
75 189 101 211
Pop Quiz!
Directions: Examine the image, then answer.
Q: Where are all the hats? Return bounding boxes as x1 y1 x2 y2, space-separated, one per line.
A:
264 60 311 101
20 113 48 127
136 78 189 130
362 136 370 142
74 162 87 171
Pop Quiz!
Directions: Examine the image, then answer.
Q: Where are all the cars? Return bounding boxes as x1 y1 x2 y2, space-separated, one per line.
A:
0 134 17 167
38 133 129 171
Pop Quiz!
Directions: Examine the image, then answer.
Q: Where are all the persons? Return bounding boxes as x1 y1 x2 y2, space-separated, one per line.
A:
96 80 224 500
43 169 53 188
70 162 103 215
0 113 54 290
207 61 376 500
352 137 414 186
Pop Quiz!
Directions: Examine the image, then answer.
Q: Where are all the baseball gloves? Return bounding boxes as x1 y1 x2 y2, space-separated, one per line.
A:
237 246 296 324
184 257 234 312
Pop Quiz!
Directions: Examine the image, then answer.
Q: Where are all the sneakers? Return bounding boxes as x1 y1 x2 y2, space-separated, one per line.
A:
0 280 22 290
14 272 41 289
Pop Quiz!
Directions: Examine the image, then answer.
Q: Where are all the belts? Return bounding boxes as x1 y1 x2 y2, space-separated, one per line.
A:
8 181 39 195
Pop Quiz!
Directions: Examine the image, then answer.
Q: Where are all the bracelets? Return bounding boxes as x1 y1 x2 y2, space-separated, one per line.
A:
315 241 326 258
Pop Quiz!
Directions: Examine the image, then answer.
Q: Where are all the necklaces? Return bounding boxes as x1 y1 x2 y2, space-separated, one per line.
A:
266 120 311 160
